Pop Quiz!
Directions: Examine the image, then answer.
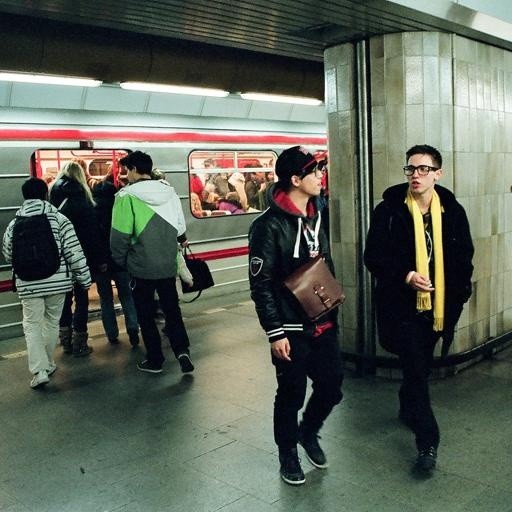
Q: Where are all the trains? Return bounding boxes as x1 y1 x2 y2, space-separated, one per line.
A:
0 121 330 298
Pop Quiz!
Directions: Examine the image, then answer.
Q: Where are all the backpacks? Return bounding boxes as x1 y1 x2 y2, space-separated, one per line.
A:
13 213 61 282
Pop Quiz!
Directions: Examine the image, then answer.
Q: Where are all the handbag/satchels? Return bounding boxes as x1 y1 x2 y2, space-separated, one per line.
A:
282 254 346 321
180 243 214 294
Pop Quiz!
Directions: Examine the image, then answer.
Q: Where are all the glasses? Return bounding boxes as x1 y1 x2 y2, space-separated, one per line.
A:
402 164 438 177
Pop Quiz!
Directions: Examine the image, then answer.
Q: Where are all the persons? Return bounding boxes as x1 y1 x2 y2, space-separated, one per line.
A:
2 151 194 387
362 145 476 478
248 147 347 487
190 157 274 222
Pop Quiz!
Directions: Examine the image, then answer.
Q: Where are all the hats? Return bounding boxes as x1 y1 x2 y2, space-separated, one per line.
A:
275 146 329 181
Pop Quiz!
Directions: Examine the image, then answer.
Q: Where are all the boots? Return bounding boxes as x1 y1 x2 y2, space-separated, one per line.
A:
58 325 71 355
71 332 95 358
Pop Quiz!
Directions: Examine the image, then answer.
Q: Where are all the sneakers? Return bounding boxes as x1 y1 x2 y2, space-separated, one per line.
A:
30 361 58 388
174 351 195 374
108 334 121 344
135 358 162 373
415 437 439 472
397 405 423 434
296 423 329 468
277 443 305 487
129 329 140 346
